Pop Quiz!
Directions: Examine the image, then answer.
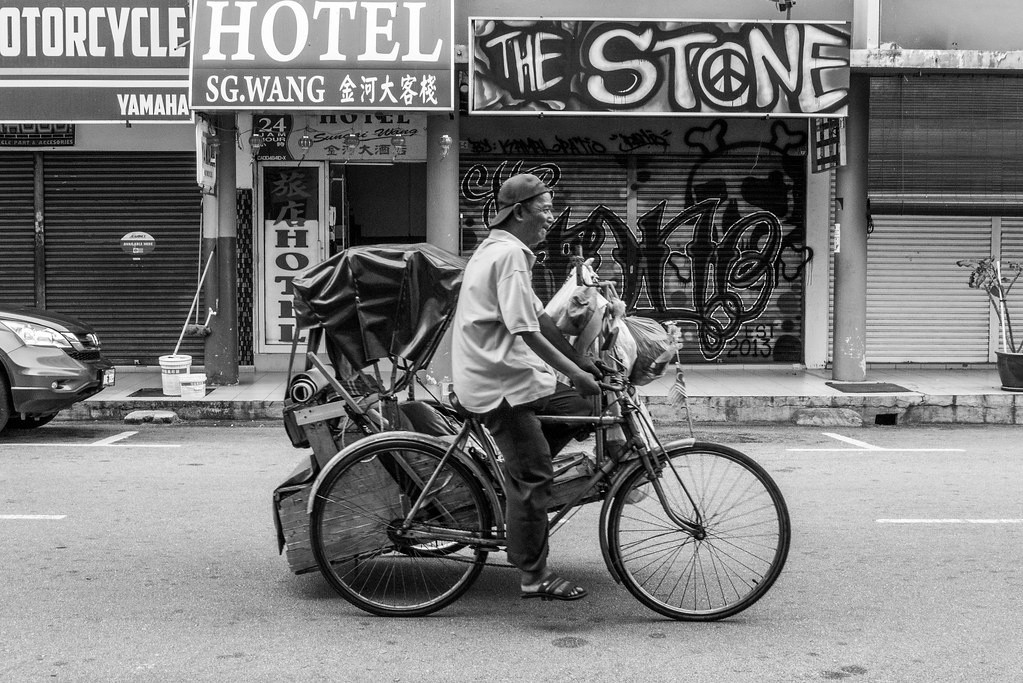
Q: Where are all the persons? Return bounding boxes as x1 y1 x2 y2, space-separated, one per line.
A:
450 175 606 603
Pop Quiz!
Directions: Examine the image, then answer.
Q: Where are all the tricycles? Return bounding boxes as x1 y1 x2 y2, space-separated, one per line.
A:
271 244 791 614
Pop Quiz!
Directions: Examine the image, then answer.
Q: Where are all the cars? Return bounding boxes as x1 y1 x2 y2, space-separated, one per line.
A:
0 303 115 436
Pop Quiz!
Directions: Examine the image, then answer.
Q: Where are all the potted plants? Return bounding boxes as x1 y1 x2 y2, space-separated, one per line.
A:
955 255 1023 393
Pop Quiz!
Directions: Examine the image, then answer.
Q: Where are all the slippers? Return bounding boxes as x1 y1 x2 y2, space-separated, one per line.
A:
520 572 589 600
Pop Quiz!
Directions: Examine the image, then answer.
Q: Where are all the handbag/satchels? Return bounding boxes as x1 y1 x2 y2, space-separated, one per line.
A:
621 313 673 387
544 265 594 333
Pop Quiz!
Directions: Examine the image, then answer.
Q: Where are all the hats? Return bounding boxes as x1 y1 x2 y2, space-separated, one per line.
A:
487 172 550 228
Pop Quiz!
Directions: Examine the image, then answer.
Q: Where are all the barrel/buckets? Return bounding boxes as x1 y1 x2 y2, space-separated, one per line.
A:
159 355 207 398
